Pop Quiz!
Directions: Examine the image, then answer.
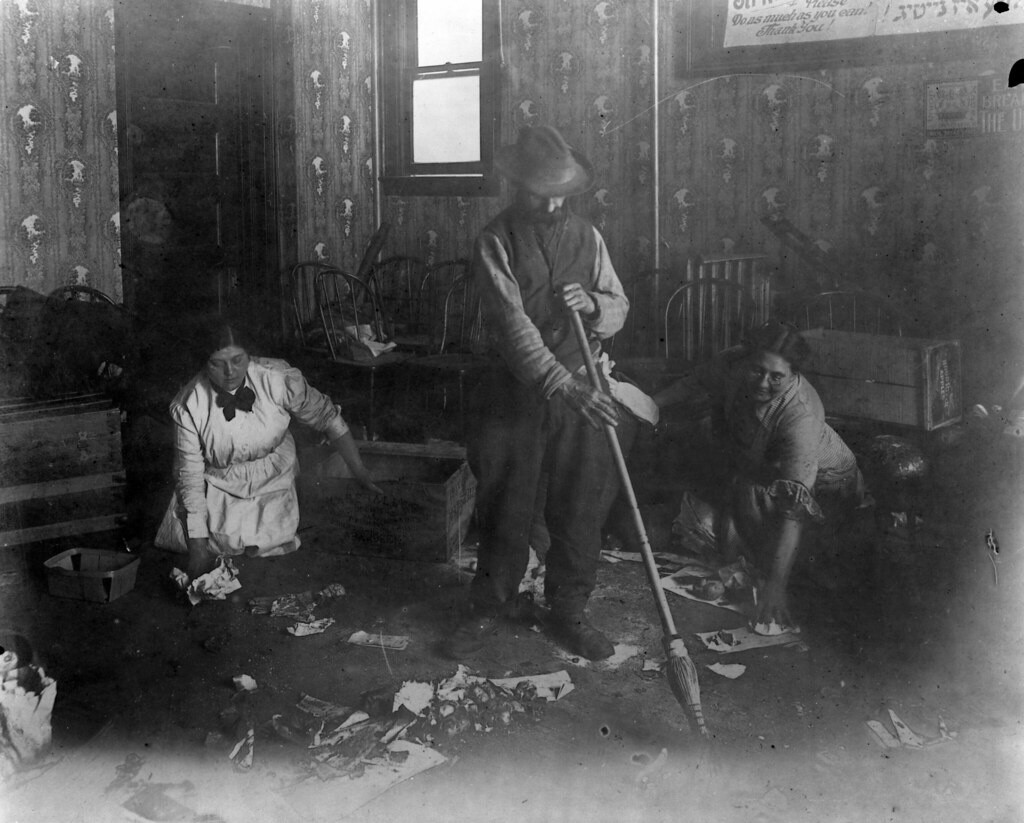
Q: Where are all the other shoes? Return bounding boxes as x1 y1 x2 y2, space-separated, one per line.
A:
448 609 503 653
543 610 613 660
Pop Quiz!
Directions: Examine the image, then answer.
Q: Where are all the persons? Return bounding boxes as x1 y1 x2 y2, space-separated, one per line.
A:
439 124 631 660
68 320 384 747
613 321 865 631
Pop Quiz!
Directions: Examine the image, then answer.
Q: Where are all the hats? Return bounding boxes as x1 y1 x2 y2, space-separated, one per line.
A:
494 122 595 198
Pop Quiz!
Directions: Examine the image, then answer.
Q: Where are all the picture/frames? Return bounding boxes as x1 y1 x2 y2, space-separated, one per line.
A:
682 0 1024 76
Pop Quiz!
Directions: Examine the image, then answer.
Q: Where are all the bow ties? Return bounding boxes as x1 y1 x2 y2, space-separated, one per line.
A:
209 377 257 421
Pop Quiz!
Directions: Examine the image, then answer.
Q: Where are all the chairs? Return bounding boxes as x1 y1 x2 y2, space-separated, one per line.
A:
416 258 474 336
422 271 513 442
314 270 429 444
644 276 757 495
369 254 432 405
789 290 904 336
288 260 388 372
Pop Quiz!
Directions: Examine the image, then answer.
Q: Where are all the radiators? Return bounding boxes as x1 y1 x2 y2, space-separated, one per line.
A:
684 253 770 365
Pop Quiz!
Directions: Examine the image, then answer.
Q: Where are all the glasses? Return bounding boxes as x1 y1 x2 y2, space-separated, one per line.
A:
749 369 784 387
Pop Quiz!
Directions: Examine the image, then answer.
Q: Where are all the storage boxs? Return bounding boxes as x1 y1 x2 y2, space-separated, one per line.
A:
43 548 139 602
295 439 482 564
796 326 963 432
